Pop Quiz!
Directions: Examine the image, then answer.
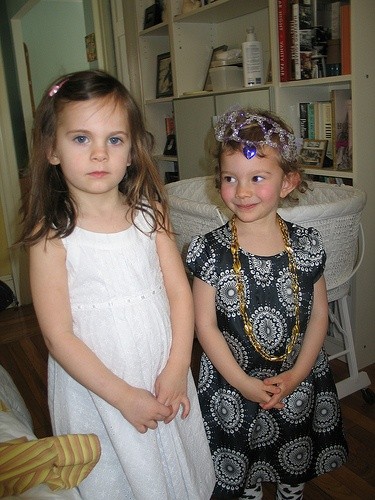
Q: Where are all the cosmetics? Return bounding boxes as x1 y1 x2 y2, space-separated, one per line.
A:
242 25 264 87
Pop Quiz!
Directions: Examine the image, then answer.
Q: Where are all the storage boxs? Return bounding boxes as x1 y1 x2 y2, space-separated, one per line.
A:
209 65 244 91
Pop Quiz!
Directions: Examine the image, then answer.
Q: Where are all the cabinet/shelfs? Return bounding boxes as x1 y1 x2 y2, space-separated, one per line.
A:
122 0 375 371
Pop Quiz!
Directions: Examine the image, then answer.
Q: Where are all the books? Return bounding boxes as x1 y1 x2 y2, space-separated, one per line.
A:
278 0 352 82
310 175 352 186
300 89 352 173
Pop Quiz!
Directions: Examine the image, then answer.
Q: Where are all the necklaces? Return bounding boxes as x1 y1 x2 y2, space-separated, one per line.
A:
230 213 300 363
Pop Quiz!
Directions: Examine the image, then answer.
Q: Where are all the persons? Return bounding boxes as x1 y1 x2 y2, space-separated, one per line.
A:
163 63 173 89
185 108 347 500
9 69 218 500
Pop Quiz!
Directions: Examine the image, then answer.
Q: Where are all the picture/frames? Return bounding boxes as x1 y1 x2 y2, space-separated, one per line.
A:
298 138 328 169
156 51 172 98
